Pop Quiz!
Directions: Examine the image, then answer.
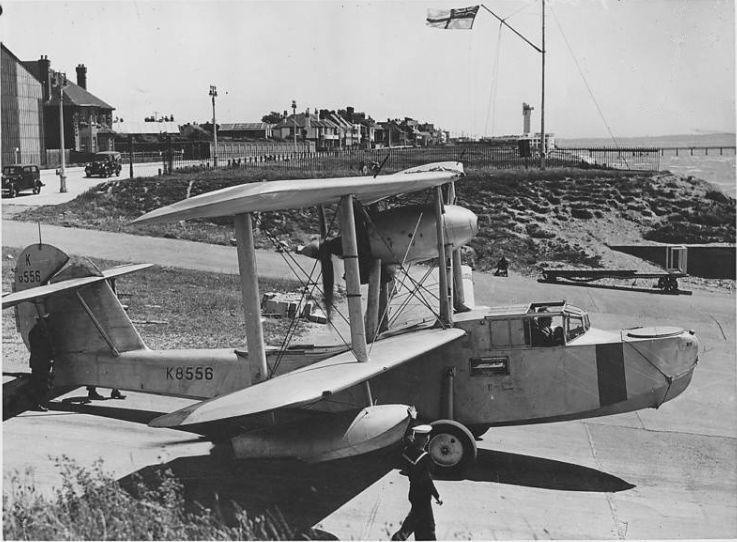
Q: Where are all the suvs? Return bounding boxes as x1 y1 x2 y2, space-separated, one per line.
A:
84 151 122 178
1 164 43 198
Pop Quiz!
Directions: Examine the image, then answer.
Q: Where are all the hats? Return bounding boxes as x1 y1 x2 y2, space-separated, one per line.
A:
413 425 432 436
34 313 50 320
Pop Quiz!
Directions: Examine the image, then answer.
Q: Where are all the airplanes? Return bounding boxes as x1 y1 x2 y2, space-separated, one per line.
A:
3 160 699 482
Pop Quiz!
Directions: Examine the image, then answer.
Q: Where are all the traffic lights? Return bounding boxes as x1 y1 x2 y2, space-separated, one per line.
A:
62 73 67 87
49 70 58 88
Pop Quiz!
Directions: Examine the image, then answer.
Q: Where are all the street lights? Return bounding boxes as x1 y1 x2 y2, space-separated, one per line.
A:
292 101 297 159
209 84 219 167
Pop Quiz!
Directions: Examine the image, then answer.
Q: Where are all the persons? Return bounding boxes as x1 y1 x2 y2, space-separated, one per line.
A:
533 306 562 347
391 425 444 541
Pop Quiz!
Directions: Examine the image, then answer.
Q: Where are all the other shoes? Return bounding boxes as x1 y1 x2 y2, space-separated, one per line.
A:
111 391 127 398
32 404 48 411
88 391 106 400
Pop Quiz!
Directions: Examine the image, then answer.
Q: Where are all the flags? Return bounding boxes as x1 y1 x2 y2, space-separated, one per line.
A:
426 5 480 30
